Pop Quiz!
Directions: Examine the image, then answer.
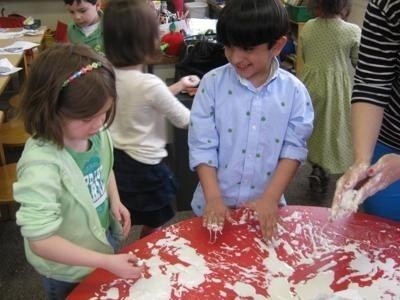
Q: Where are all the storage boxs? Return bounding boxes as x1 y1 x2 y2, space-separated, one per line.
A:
283 1 315 22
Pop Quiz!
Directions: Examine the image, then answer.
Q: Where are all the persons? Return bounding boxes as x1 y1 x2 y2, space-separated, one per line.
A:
63 0 103 56
12 43 144 299
330 1 400 222
299 0 361 209
188 0 315 246
102 1 200 241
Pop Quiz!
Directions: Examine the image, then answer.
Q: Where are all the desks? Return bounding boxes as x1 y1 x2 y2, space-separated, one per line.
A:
0 25 48 219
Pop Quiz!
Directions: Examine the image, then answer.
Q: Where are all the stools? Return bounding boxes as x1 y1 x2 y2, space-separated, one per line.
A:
0 119 31 166
0 162 17 218
